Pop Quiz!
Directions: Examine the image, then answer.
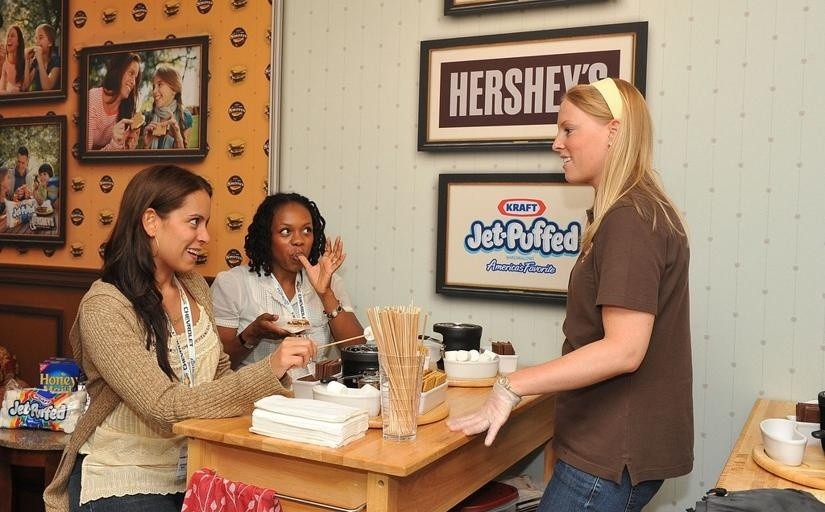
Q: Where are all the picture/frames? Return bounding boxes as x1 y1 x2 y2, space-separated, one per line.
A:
0 0 72 104
79 32 210 165
0 114 69 249
415 19 651 154
433 171 596 303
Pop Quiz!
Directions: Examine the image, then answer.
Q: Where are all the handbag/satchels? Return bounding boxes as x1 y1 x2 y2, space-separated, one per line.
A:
695 487 825 512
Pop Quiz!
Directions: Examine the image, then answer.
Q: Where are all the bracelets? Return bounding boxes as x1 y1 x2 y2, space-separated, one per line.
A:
492 373 522 408
323 304 344 327
237 333 255 351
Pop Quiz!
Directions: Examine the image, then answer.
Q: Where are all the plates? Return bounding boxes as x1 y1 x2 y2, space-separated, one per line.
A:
34 206 54 217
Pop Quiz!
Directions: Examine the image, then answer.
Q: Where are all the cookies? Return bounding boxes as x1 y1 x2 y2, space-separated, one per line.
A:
297 358 341 381
420 368 447 393
795 403 821 422
492 341 515 355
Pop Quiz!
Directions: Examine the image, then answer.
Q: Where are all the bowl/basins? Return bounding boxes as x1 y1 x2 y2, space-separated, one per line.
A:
760 418 808 466
313 383 382 418
440 354 500 380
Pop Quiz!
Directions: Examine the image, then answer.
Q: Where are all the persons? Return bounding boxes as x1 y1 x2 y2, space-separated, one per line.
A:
33 163 57 199
85 48 145 154
208 191 370 380
0 22 23 98
8 145 35 203
442 74 694 510
140 64 192 148
1 167 17 221
66 165 320 510
24 20 58 91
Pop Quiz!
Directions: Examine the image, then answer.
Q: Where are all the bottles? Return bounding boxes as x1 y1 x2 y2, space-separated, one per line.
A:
359 367 382 389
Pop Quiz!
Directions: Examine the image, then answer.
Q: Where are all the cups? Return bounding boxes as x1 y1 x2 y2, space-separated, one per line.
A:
376 349 425 440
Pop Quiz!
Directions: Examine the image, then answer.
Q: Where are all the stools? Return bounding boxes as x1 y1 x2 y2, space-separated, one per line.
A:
0 425 71 512
